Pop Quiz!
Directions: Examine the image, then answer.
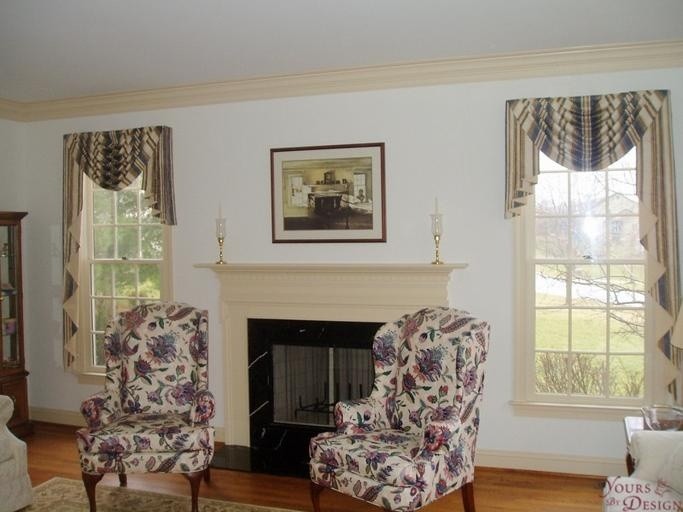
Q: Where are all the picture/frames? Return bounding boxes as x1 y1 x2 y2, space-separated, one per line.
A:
265 142 387 244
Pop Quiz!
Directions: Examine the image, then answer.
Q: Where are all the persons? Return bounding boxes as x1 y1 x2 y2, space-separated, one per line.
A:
356 189 365 201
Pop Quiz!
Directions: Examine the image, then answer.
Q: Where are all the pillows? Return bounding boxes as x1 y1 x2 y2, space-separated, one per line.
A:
605 474 682 511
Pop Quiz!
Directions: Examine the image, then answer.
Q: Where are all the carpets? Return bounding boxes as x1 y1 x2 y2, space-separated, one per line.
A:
10 475 304 512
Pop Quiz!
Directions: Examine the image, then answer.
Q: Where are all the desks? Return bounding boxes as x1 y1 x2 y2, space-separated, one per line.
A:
620 415 683 477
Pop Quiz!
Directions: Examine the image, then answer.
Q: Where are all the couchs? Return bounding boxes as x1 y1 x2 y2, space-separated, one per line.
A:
601 428 682 511
68 298 217 511
304 301 492 512
0 394 36 511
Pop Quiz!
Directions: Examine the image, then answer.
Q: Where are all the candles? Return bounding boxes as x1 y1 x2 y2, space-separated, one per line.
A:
426 193 442 237
211 201 226 240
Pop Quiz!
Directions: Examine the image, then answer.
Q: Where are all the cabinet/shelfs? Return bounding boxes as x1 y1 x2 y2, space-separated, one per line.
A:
0 208 32 442
